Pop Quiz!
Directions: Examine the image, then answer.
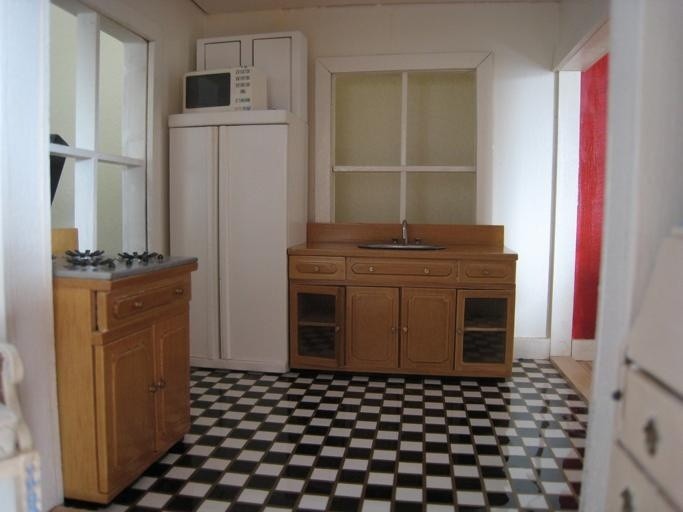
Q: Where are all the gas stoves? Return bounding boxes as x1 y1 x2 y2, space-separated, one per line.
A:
52 249 197 279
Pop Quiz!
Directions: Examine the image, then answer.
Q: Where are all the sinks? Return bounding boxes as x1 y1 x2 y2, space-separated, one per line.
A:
356 242 447 251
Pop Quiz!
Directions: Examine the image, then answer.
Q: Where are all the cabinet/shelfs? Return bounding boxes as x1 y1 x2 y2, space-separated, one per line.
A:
52 256 199 506
194 32 307 115
599 227 682 511
165 114 306 375
285 250 518 378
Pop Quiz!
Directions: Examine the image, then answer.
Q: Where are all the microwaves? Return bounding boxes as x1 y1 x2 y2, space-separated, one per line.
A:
182 66 267 112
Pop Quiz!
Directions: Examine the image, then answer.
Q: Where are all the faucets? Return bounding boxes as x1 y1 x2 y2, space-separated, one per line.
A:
401 219 409 244
